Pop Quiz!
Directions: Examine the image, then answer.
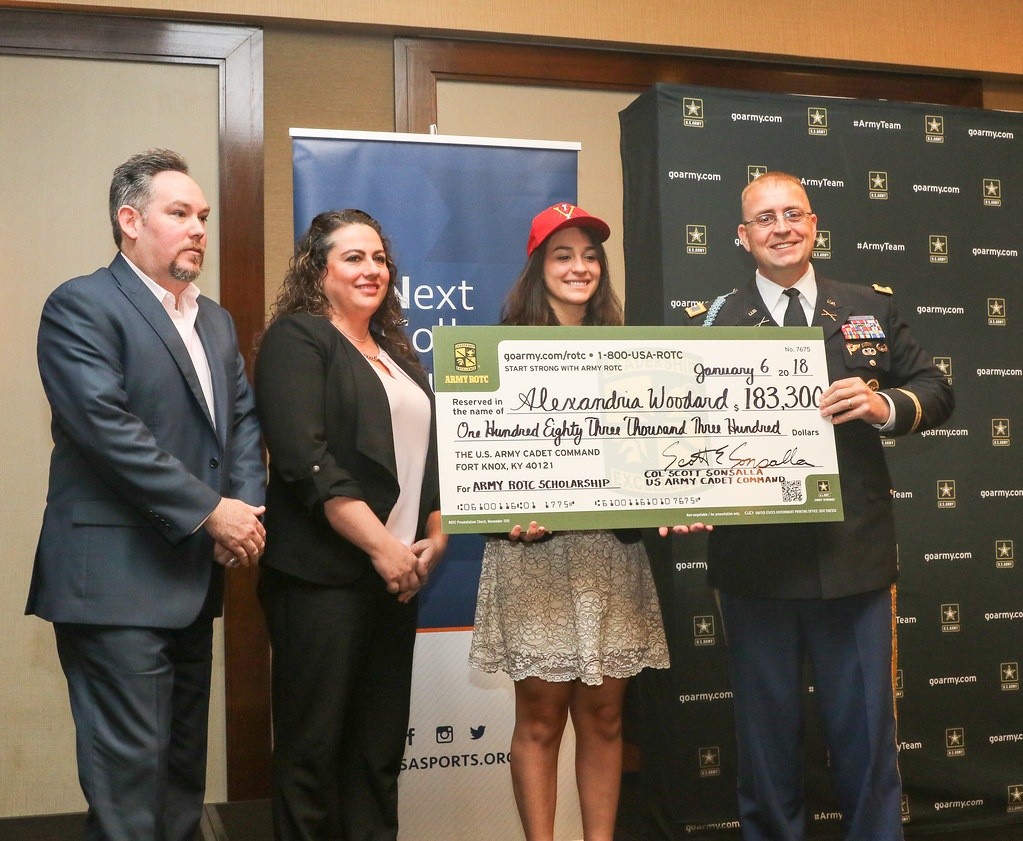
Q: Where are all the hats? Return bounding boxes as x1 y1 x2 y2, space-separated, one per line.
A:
527 202 610 256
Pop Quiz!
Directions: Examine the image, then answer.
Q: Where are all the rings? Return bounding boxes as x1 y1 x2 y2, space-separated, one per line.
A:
848 398 852 409
229 558 238 565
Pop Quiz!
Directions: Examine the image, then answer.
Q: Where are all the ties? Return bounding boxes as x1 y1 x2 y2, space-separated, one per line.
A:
782 287 808 326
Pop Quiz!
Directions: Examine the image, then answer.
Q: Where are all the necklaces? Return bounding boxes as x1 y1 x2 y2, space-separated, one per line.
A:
363 353 377 362
331 321 370 342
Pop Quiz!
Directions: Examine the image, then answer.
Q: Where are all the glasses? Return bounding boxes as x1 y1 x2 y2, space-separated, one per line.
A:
743 210 812 227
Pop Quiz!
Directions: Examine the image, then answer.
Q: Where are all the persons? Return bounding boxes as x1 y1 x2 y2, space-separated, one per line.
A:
660 173 955 841
24 151 267 841
469 200 671 840
252 207 449 841
